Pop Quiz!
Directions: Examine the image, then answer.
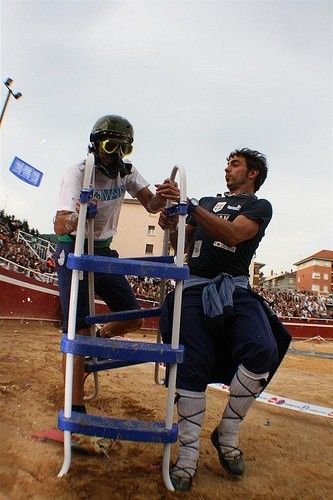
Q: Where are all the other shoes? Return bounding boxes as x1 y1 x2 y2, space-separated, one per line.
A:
170 457 199 491
211 426 245 475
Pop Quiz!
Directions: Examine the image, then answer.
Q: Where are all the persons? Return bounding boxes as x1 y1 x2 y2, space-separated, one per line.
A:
55 114 178 415
158 148 276 491
0 215 333 319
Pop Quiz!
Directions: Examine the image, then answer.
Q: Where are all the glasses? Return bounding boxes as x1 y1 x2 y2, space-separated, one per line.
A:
97 138 133 156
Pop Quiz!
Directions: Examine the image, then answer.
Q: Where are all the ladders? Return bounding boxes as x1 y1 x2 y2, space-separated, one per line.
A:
56 152 191 493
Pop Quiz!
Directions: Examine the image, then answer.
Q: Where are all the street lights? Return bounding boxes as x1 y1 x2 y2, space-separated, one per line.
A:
0 77 22 126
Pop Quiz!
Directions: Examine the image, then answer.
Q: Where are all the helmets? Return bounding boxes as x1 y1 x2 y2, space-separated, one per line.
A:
89 114 134 143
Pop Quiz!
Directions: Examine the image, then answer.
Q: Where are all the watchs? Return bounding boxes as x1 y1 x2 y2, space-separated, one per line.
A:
188 198 199 214
